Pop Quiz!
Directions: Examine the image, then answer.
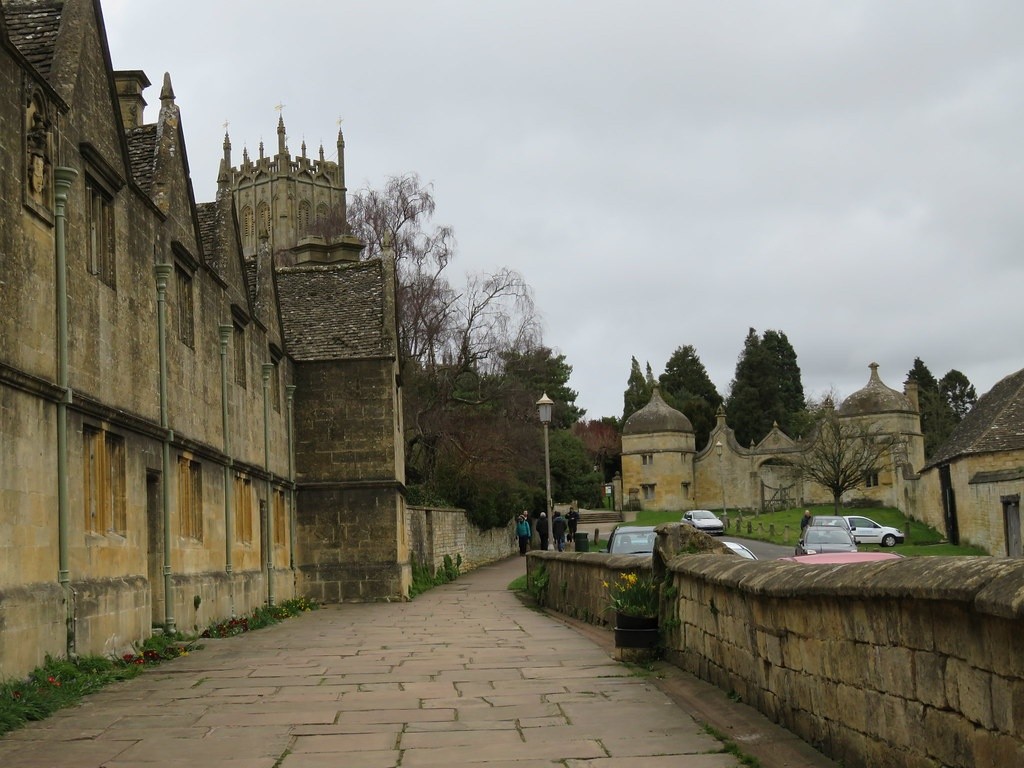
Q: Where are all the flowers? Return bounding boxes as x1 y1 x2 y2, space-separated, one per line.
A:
595 570 657 617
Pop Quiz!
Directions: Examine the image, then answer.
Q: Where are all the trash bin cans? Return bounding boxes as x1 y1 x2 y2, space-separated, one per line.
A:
575 532 590 552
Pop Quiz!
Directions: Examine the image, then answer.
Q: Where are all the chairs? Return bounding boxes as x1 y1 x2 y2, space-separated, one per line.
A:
648 534 657 544
620 535 631 545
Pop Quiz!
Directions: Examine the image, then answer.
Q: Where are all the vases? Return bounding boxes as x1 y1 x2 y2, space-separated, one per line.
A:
616 613 660 629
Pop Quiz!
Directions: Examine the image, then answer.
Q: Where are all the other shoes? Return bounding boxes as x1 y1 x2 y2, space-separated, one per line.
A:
521 553 525 557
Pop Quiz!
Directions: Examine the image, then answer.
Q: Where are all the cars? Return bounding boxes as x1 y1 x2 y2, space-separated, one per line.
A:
829 515 904 547
795 524 860 555
681 510 725 536
725 541 758 559
807 516 856 543
778 551 906 564
598 524 658 557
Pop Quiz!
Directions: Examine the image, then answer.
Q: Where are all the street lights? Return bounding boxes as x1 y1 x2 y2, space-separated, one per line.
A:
536 391 555 550
716 441 727 520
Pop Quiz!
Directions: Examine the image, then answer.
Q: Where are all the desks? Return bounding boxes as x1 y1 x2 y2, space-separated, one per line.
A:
613 629 659 661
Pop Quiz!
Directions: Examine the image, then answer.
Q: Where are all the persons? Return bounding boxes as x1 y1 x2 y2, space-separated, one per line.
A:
801 510 811 532
536 511 549 551
564 507 579 537
552 511 567 551
515 510 532 557
